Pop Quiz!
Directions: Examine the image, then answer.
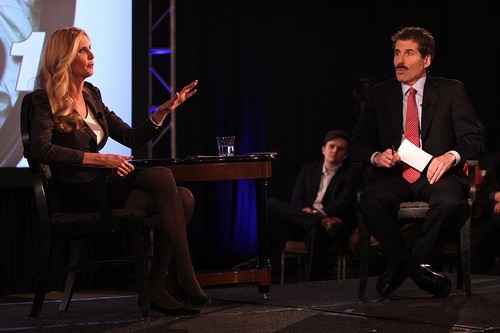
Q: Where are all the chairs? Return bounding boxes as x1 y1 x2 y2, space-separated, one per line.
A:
352 160 478 299
19 94 150 320
280 240 345 285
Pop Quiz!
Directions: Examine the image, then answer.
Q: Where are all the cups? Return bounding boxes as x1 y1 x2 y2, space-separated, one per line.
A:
217 136 235 156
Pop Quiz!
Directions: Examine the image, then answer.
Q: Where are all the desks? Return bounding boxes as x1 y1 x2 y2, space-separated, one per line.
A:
170 160 272 293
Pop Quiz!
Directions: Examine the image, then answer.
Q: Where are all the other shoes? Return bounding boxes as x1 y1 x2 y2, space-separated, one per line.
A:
322 216 343 237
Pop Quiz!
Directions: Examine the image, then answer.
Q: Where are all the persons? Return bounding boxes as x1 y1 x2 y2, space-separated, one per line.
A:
27 27 211 317
266 130 358 282
358 25 500 299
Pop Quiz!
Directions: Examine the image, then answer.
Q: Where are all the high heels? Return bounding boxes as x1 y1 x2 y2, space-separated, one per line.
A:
138 293 201 316
165 273 211 306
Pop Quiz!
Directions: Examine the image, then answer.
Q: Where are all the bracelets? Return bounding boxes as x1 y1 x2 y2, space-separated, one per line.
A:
150 115 162 127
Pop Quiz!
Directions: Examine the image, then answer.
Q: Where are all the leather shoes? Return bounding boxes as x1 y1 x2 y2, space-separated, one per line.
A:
408 263 453 298
375 262 409 296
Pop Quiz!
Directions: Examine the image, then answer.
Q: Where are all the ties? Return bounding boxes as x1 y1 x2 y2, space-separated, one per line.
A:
402 87 421 184
